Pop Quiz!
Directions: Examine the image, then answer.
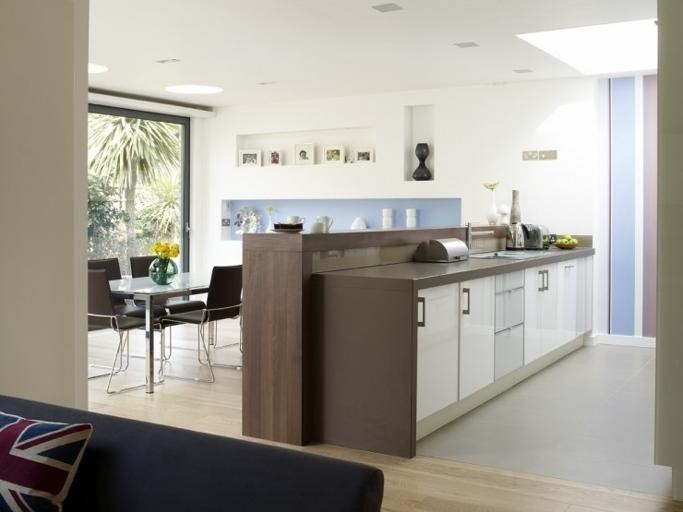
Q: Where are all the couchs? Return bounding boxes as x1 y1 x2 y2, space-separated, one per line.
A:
0 394 385 512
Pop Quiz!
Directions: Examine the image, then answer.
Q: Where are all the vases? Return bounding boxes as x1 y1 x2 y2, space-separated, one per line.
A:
510 190 521 225
149 258 178 285
265 216 273 233
487 189 497 224
412 143 432 181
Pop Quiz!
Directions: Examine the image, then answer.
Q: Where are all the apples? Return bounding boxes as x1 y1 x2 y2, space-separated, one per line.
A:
557 239 562 244
561 239 568 245
570 238 578 244
564 234 572 240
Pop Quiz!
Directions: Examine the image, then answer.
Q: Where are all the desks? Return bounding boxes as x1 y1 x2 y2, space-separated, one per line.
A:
109 272 210 393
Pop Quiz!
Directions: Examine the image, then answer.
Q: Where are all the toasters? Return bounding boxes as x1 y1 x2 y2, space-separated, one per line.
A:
524 224 542 248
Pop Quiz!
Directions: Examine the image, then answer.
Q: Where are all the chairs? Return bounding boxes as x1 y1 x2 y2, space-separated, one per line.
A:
130 256 205 360
159 264 243 383
88 269 164 394
89 258 172 372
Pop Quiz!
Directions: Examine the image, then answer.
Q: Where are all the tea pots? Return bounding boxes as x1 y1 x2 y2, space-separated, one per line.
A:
312 216 332 232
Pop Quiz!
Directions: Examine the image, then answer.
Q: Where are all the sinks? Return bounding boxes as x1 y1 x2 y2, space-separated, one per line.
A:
467 250 550 260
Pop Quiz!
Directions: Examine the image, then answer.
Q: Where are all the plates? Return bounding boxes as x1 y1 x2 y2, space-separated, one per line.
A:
273 229 304 233
553 242 578 248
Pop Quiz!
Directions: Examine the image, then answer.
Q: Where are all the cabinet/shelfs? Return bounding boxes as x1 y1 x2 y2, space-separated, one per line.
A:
495 324 524 380
459 275 495 401
494 288 525 333
496 269 525 294
417 282 460 423
525 256 594 365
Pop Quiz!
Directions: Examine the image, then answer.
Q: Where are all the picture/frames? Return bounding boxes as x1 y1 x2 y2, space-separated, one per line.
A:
323 146 346 164
239 149 261 167
293 143 316 164
355 149 374 162
269 152 281 166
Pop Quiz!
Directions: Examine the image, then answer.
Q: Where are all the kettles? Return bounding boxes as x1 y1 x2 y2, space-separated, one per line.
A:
505 221 528 250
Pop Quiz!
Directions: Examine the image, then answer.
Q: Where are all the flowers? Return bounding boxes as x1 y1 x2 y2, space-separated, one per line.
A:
482 181 499 191
265 205 274 216
148 241 180 284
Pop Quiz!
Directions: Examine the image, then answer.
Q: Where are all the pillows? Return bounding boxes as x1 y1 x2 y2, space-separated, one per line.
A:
0 411 92 512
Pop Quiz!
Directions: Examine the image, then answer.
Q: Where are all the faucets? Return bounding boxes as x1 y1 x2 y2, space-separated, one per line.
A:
466 222 495 250
